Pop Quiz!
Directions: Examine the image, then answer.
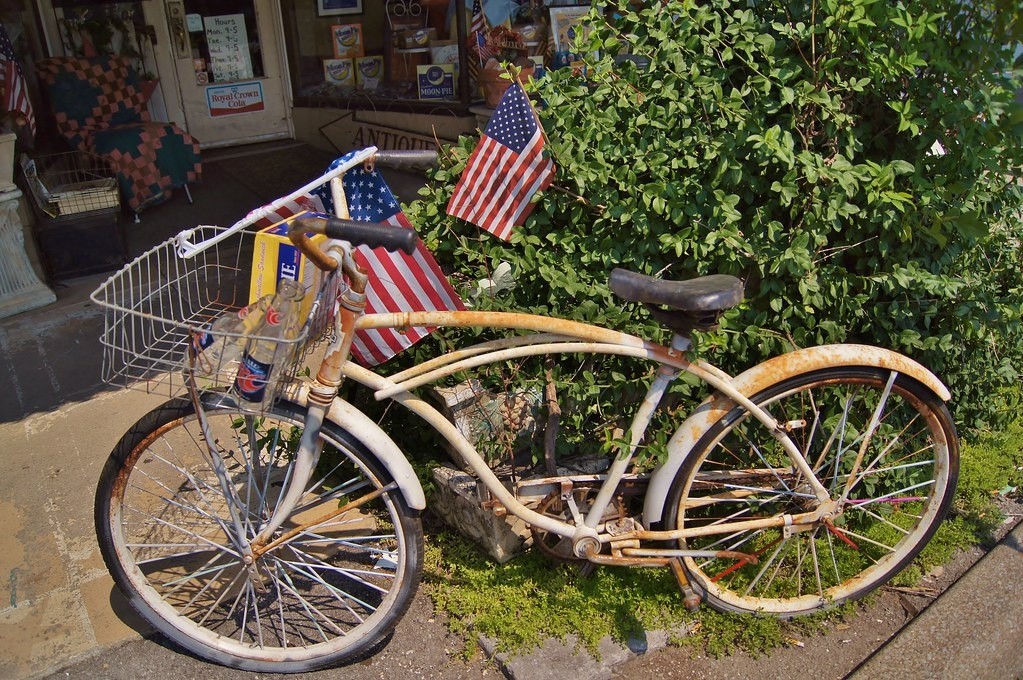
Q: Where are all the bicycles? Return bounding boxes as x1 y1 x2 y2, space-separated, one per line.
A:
89 147 960 673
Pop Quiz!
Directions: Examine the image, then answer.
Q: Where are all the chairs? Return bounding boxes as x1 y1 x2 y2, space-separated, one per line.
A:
27 55 204 225
385 0 432 83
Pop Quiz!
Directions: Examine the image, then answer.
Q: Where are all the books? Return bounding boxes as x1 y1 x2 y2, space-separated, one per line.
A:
20 153 56 219
49 178 121 216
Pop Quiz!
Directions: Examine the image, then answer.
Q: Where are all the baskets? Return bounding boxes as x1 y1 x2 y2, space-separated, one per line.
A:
90 225 338 415
20 149 120 219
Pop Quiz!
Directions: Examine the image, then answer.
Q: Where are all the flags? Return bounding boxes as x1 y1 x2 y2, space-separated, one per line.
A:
445 82 556 242
470 0 484 43
473 34 498 60
240 150 467 369
0 22 36 145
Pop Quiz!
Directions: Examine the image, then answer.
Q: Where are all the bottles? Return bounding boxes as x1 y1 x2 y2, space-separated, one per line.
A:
185 280 305 412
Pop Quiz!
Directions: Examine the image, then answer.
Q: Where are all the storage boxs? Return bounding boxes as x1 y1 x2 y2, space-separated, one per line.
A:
320 24 461 100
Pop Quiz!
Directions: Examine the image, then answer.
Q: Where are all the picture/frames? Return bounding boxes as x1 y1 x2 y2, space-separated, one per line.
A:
317 0 363 17
